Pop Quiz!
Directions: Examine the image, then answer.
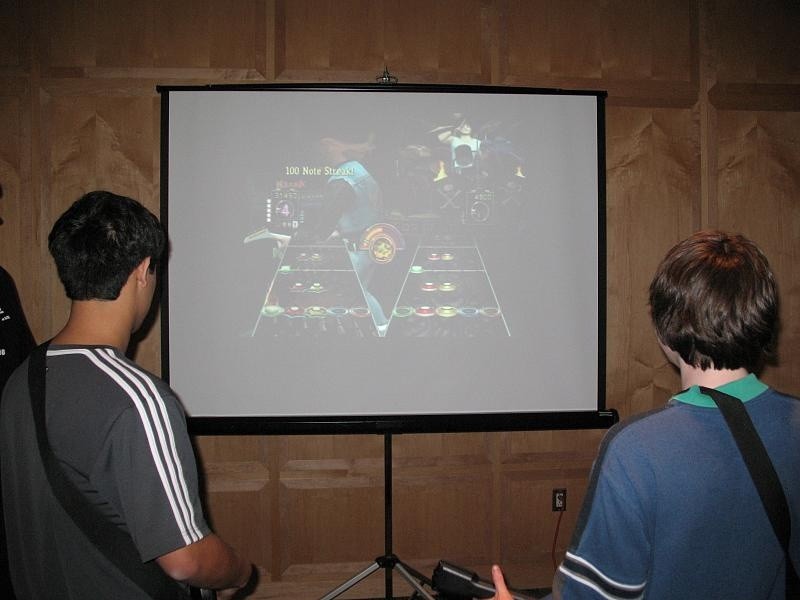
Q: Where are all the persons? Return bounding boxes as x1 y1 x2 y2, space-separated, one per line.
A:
0 190 252 600
480 231 799 600
0 181 37 600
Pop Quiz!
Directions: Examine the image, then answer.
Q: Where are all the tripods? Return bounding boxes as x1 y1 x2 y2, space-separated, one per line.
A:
317 434 439 600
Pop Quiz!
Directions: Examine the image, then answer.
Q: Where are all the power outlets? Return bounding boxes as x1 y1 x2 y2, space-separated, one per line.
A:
556 493 563 508
